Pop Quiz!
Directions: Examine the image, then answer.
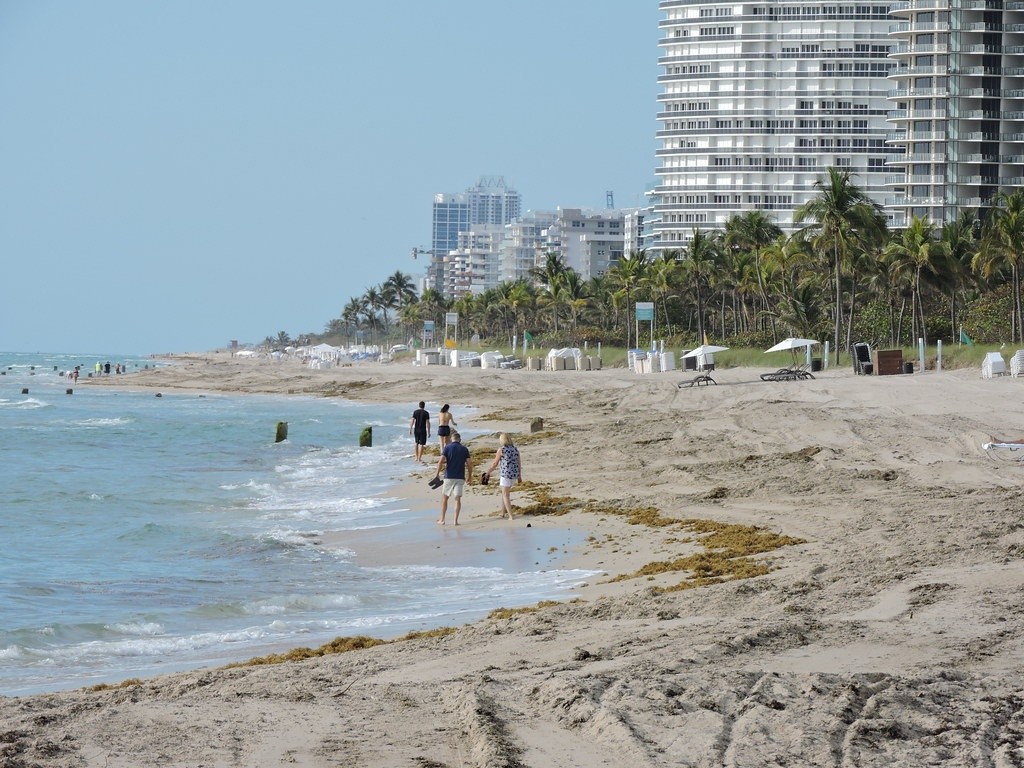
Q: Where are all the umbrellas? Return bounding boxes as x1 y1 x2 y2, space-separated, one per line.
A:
763 337 819 369
284 347 306 355
313 343 336 353
680 345 729 383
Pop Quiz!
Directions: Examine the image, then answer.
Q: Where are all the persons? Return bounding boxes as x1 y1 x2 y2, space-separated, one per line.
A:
437 404 457 453
104 361 110 376
114 362 121 374
409 401 430 462
95 361 102 376
485 433 522 521
65 367 79 384
436 433 472 526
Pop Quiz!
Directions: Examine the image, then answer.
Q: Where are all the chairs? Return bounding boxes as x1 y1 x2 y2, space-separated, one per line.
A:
758 363 815 381
981 350 1023 379
852 341 874 375
677 367 718 389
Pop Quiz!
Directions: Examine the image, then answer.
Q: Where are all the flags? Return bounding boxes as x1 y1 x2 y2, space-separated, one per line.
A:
703 333 708 345
444 339 455 348
961 331 975 349
525 331 532 342
412 339 420 347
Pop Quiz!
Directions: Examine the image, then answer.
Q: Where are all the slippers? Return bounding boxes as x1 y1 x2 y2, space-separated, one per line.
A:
428 474 443 489
481 472 490 485
